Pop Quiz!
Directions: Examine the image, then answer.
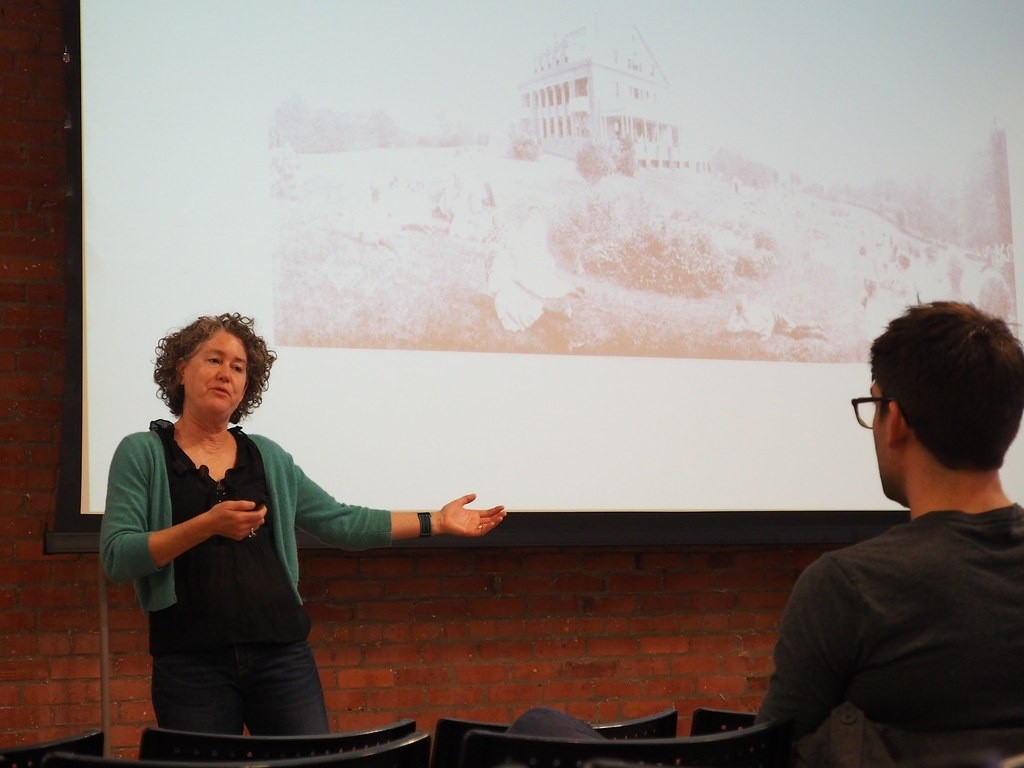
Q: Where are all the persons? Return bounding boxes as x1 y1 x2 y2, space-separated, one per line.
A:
99 311 509 735
501 298 1024 767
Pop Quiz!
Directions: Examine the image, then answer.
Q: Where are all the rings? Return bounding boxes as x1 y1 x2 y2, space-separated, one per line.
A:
478 525 482 530
248 527 257 537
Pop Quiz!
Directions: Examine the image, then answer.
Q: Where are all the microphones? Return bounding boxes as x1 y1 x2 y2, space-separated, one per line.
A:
199 465 209 480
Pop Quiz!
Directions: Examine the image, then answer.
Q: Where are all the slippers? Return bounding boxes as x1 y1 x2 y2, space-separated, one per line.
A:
852 396 914 429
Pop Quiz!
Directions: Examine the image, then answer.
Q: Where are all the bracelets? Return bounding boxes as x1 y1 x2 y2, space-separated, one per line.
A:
416 512 432 537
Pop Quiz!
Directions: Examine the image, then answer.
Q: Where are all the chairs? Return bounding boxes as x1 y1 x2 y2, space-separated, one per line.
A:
0 708 1024 768
